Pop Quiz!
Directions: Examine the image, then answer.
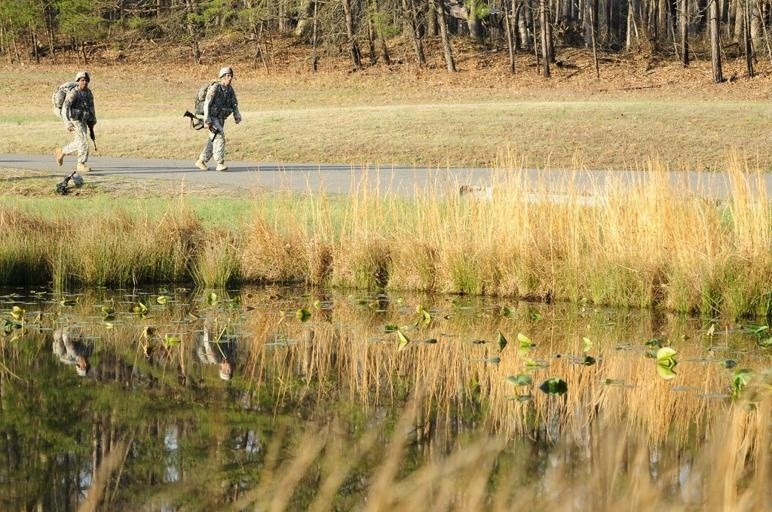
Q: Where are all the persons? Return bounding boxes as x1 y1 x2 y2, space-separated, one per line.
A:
61 327 95 376
203 306 237 383
54 72 96 172
194 67 243 171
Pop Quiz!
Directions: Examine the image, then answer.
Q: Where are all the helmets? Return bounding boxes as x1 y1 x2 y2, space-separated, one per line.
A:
75 71 90 82
218 67 233 78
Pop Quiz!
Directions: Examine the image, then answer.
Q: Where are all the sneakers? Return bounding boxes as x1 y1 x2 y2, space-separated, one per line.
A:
194 160 208 171
77 164 91 172
216 165 227 170
55 147 63 166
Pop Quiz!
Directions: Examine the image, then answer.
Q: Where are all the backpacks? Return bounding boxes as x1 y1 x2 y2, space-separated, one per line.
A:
52 82 90 118
194 80 222 114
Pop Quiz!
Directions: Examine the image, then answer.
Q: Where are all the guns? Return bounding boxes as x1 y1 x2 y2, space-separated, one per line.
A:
56 171 76 194
183 111 221 142
88 125 97 151
